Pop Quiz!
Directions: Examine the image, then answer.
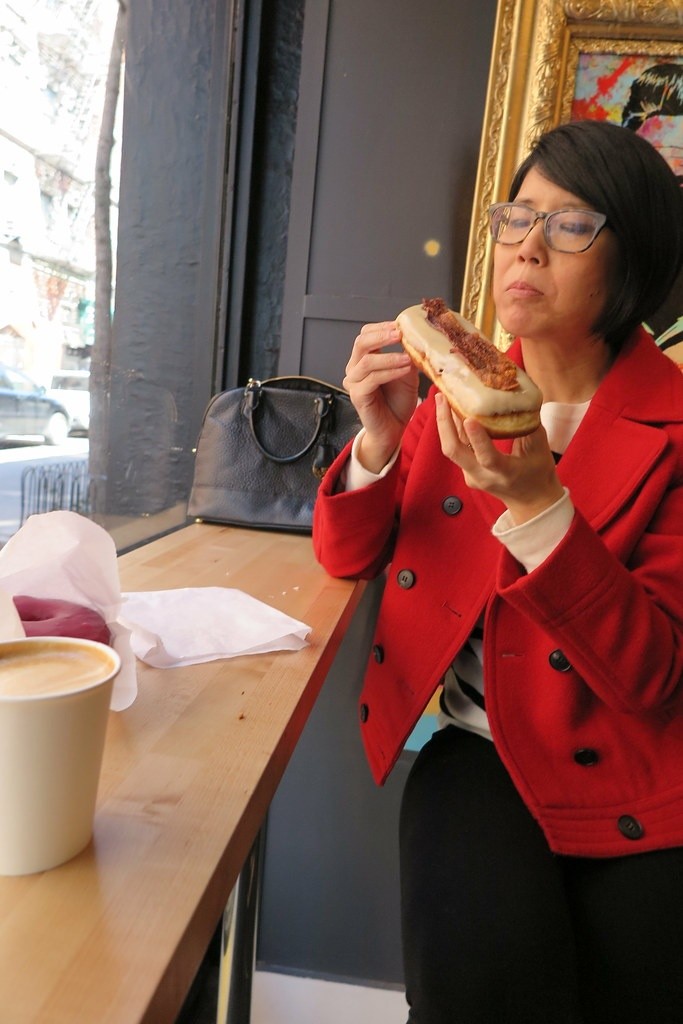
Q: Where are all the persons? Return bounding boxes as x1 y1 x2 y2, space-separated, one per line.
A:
311 121 683 1023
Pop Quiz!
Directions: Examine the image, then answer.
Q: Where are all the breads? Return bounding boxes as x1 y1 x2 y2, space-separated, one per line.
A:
395 298 543 440
9 595 111 650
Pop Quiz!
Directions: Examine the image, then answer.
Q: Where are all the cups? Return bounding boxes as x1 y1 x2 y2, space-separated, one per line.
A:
0 636 122 877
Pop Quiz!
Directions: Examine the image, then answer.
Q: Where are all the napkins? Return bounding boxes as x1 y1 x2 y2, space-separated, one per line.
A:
119 586 312 668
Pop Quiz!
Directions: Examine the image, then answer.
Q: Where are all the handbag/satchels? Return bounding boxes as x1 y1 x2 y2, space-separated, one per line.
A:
186 376 365 531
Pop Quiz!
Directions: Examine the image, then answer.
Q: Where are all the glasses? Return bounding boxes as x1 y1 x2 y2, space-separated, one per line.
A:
487 202 625 255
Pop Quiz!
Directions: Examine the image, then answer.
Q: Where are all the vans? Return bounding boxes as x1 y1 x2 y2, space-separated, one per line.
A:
47 369 89 438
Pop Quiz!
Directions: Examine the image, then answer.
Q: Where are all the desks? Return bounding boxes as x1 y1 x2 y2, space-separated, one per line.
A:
0 520 375 1024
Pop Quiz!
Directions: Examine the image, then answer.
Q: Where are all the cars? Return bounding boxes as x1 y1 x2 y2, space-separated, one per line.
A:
0 365 72 446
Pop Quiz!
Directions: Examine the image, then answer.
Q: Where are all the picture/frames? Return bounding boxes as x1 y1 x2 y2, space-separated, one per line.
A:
461 0 683 366
